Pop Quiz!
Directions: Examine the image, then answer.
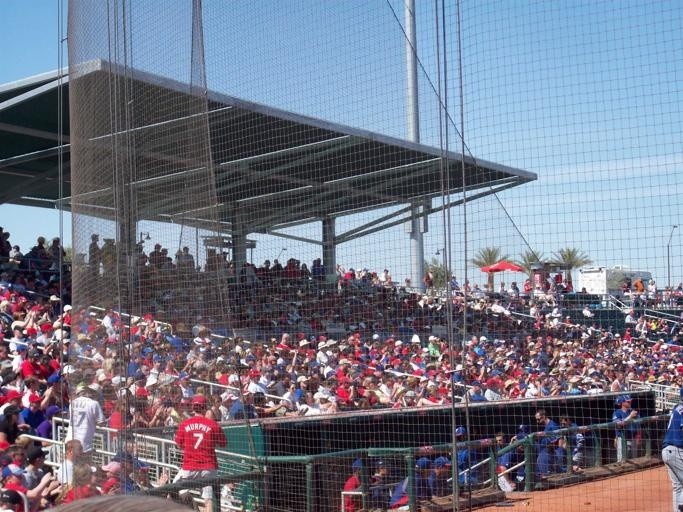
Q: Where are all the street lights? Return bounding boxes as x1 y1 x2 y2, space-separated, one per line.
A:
667 224 678 289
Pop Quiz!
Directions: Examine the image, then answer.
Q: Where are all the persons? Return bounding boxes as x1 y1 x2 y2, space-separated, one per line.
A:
0 230 683 511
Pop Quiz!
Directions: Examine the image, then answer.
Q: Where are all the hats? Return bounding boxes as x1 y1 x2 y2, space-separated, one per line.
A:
0 284 683 504
